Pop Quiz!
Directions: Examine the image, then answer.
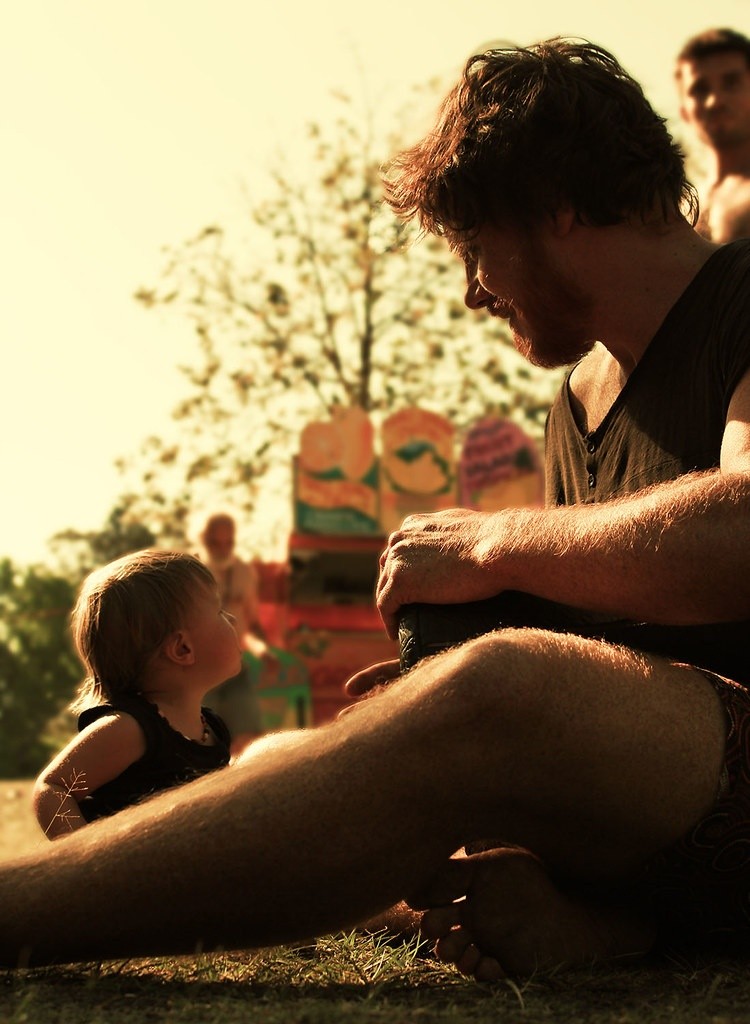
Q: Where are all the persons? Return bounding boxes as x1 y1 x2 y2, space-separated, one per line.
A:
201 514 256 634
675 27 750 243
0 34 750 982
33 545 242 840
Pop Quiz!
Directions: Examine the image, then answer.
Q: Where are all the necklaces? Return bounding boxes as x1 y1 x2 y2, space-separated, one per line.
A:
158 709 209 744
